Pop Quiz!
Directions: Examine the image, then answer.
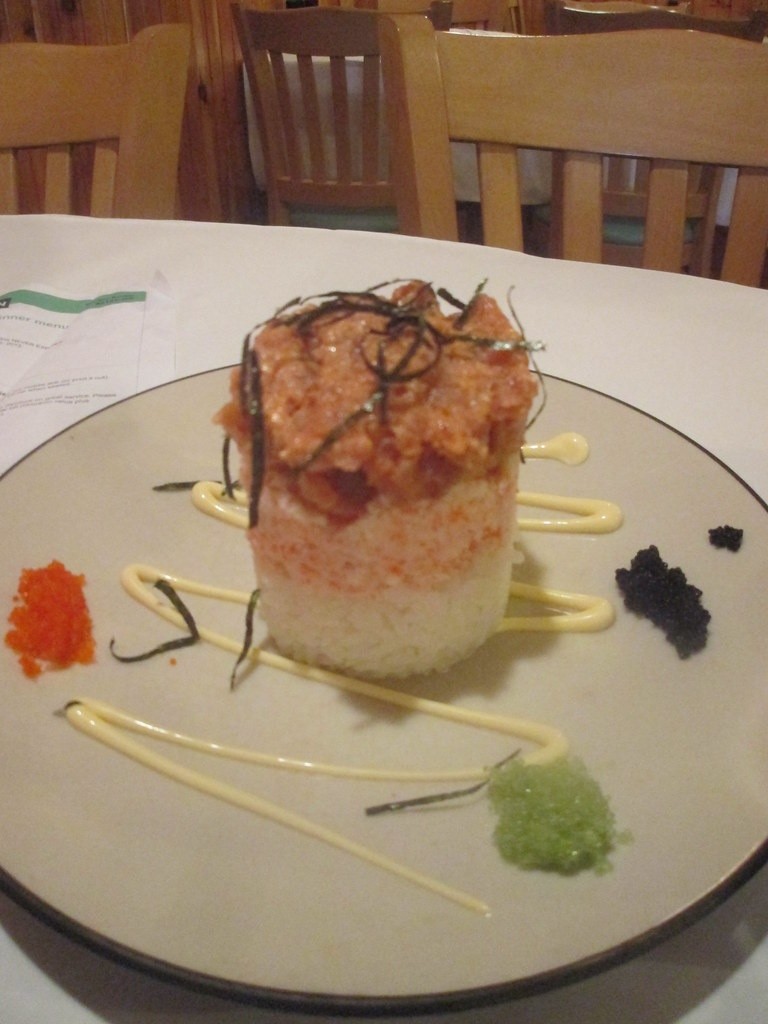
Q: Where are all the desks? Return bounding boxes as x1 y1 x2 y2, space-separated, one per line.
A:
241 29 737 226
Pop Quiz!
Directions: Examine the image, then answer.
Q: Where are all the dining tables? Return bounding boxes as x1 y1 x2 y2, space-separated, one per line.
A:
0 215 768 1024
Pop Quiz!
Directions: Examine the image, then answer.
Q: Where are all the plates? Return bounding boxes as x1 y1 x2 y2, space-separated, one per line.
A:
0 358 767 1013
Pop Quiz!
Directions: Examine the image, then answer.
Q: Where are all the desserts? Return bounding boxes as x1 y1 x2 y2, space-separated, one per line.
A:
208 279 539 686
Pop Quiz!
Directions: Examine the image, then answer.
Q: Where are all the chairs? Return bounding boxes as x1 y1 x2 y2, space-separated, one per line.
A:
0 0 768 290
